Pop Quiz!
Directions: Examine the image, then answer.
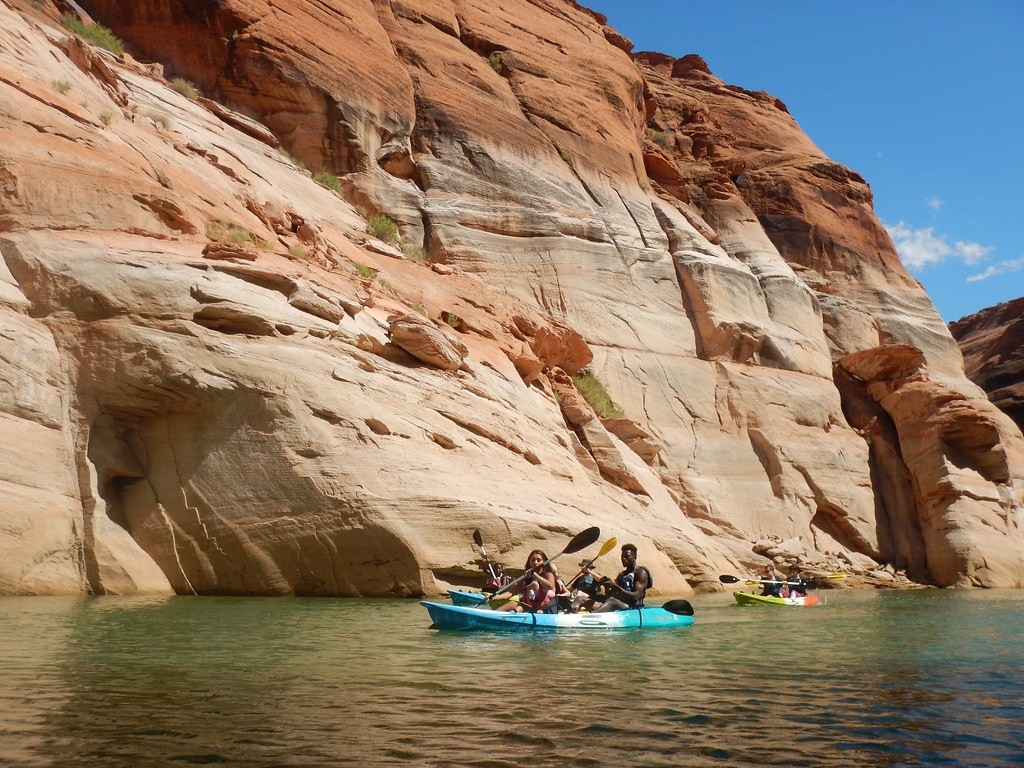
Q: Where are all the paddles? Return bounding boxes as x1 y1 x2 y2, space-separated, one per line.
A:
565 536 617 587
745 572 847 586
558 598 695 616
473 529 497 579
428 526 600 629
719 575 816 589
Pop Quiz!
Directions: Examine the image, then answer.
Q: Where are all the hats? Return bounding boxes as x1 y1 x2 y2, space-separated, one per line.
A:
578 559 595 569
788 565 801 574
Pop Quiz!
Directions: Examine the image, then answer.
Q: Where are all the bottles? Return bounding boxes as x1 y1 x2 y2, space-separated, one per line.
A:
529 590 535 599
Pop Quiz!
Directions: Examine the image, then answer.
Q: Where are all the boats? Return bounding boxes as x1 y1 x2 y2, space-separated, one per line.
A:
733 590 820 607
420 589 695 632
447 590 486 608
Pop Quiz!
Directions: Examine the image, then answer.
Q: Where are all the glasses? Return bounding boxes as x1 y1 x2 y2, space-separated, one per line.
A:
764 570 772 572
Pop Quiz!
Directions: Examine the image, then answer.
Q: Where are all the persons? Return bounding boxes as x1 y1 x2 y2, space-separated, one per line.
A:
745 564 814 598
562 558 603 600
482 564 509 595
549 561 570 597
482 550 555 613
564 543 653 614
